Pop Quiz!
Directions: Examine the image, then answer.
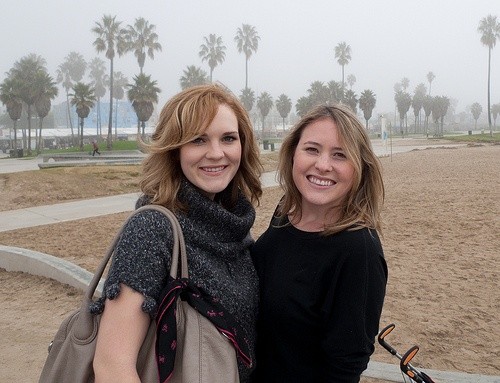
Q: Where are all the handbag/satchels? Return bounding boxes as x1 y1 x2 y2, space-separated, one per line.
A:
38 205 241 382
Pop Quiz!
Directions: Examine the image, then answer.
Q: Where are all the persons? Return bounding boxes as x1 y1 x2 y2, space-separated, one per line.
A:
426 128 430 137
92 82 262 383
53 140 73 149
2 143 6 153
490 128 493 138
17 145 21 148
92 141 101 156
249 102 390 383
481 128 484 135
